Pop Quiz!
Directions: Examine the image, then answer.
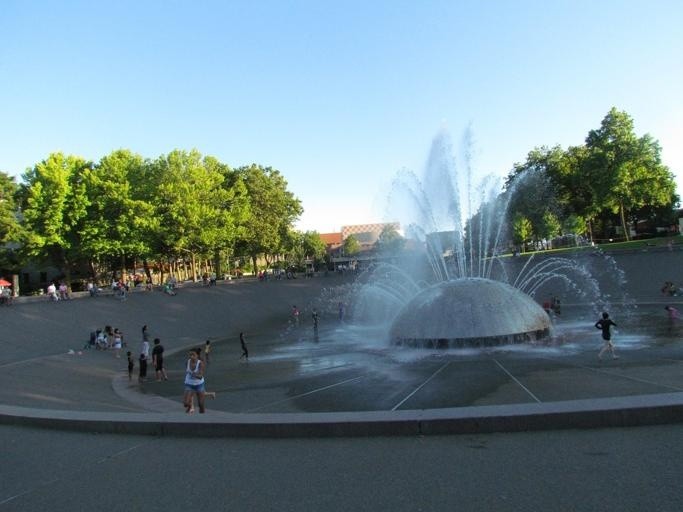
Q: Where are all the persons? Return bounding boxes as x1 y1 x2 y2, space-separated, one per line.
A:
291 301 345 329
660 281 683 297
239 332 250 361
89 325 168 383
542 291 562 315
183 348 217 413
664 305 680 336
0 273 176 306
201 271 216 288
205 339 212 362
594 311 619 361
259 269 269 281
